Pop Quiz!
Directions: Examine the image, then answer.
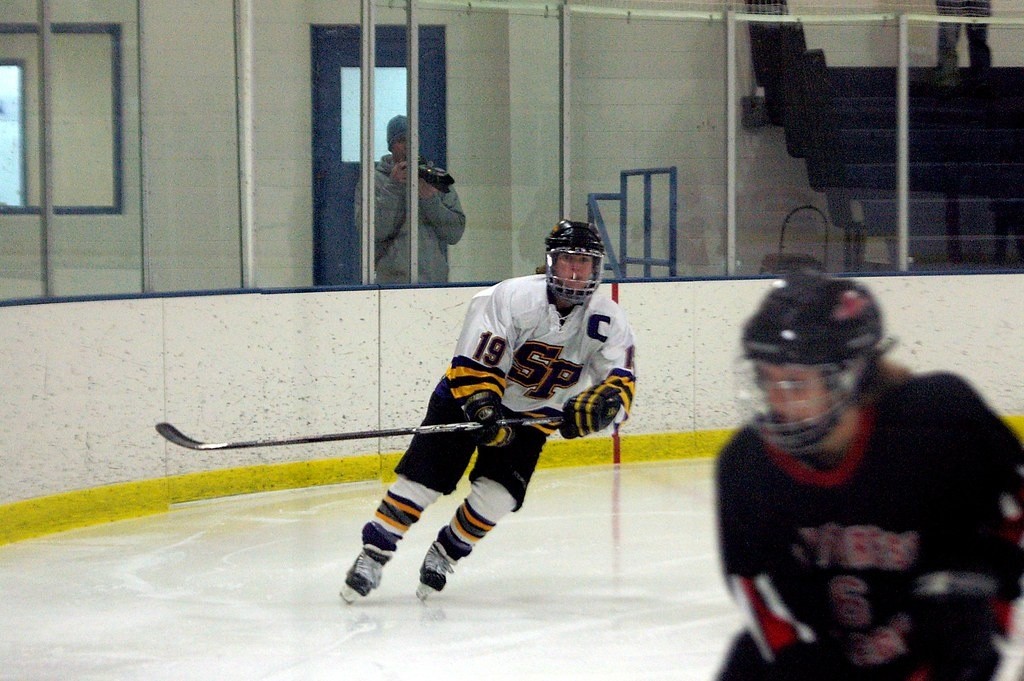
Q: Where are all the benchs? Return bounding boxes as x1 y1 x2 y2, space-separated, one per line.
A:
773 50 1024 272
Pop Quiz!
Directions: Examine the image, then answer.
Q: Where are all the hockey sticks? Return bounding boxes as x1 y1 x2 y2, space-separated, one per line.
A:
156 412 566 452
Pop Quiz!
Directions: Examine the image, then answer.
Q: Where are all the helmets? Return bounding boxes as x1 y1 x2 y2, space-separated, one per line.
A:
735 268 899 456
545 219 605 306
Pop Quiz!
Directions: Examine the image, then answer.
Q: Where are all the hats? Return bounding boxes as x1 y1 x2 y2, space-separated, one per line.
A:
387 115 407 152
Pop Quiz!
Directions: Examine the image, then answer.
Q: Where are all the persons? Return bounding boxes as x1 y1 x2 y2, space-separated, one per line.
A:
340 219 637 603
713 275 1024 681
354 115 466 285
933 0 992 87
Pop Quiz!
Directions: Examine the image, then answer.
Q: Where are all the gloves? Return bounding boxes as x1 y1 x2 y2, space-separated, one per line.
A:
910 592 1000 681
559 384 624 439
461 390 515 448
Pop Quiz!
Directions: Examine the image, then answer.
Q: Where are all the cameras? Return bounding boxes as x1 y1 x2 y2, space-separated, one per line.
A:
416 155 454 194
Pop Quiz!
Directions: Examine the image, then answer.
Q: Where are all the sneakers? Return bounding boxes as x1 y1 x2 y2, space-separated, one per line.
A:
340 543 403 603
416 541 472 602
